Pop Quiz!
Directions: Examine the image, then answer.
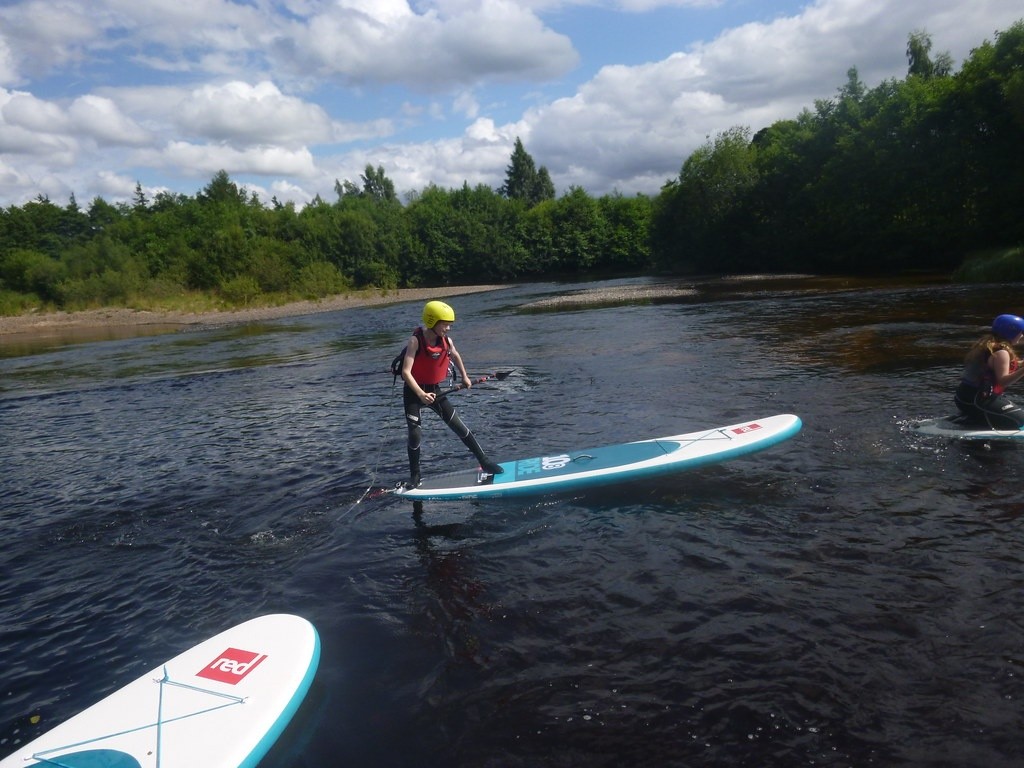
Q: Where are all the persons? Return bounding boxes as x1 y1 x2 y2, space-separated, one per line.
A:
947 314 1024 428
400 301 503 488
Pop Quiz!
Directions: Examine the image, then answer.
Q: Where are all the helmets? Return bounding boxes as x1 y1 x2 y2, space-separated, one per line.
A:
992 314 1024 339
422 301 455 329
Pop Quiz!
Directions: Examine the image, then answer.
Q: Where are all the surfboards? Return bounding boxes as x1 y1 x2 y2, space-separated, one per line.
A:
0 612 322 768
901 412 1024 440
393 412 803 501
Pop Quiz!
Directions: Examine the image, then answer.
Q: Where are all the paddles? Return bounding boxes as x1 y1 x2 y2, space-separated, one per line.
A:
430 368 518 399
